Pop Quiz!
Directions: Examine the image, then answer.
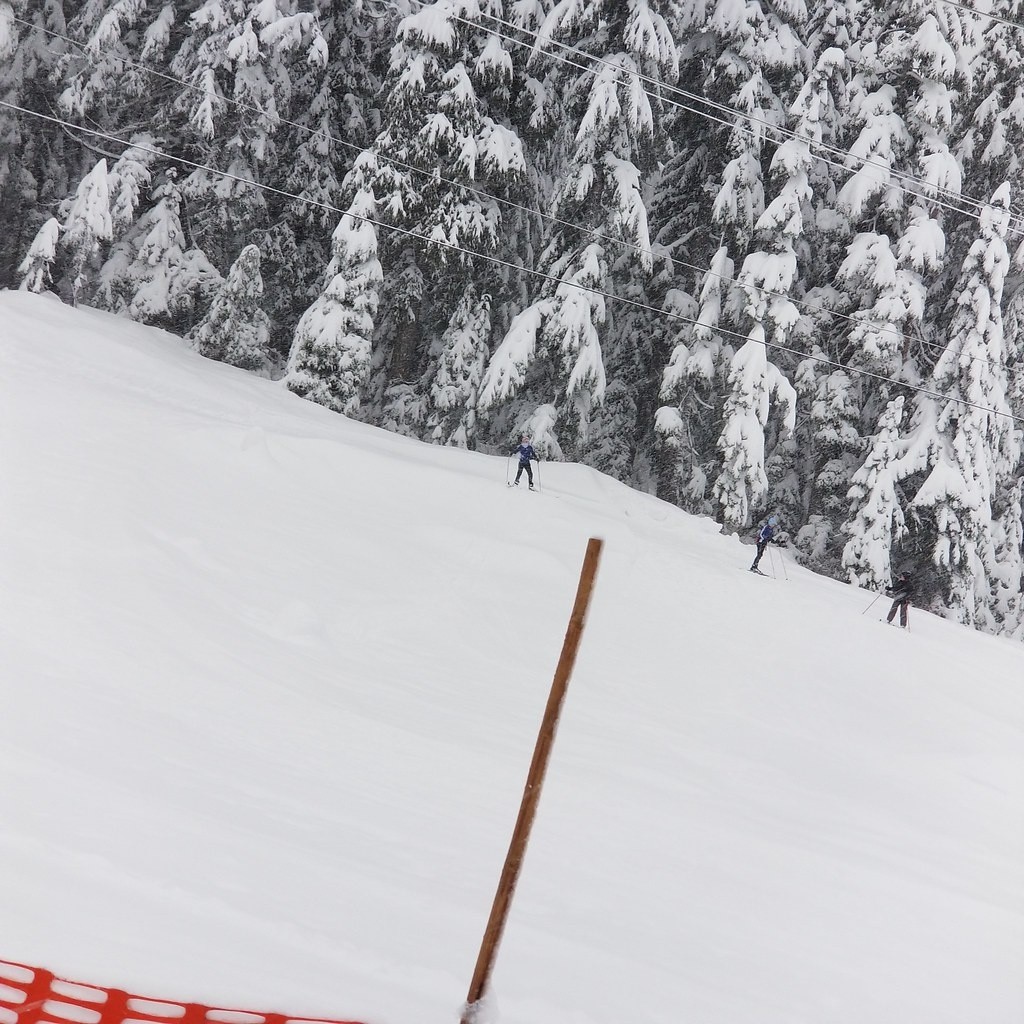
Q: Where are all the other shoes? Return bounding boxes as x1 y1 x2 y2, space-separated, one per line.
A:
751 565 761 573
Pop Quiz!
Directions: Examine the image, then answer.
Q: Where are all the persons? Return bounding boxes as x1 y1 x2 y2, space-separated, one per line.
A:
509 437 540 490
750 517 778 575
885 572 912 627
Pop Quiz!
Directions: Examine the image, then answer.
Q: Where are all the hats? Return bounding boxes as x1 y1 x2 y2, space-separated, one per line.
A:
522 437 529 442
768 518 777 526
902 571 911 579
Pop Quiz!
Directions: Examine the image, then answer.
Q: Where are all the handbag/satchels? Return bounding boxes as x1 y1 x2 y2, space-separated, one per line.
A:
755 535 761 544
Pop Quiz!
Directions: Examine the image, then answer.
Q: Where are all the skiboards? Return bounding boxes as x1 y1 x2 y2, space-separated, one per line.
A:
739 568 775 579
878 618 910 631
506 478 537 492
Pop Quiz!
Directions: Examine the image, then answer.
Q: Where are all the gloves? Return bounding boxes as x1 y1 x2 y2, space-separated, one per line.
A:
767 537 772 542
771 540 777 544
885 586 890 590
536 459 539 463
510 453 513 457
